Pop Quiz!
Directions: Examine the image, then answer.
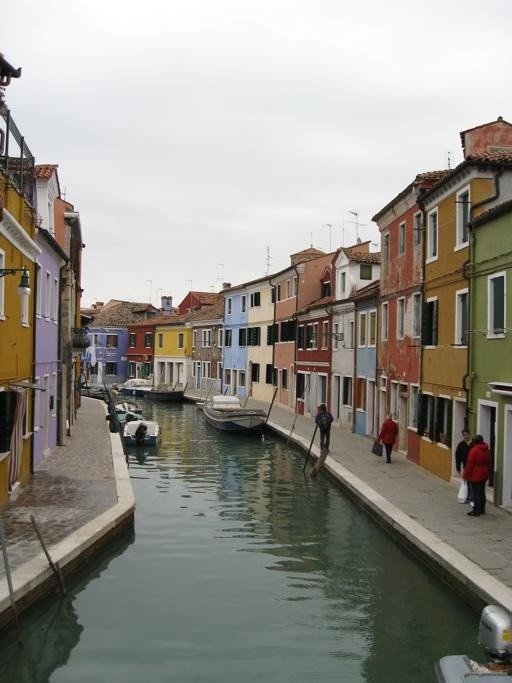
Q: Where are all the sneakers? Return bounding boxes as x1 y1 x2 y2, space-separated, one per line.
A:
467 501 485 516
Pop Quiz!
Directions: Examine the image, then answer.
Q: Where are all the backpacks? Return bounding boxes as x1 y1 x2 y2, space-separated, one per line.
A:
318 412 331 429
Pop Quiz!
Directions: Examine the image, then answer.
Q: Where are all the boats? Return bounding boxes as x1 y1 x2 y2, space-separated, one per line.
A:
116 402 159 446
433 605 511 683
203 395 266 431
119 379 184 401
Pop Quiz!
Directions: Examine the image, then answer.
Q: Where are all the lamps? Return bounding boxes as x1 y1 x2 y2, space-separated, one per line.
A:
310 333 344 344
0 265 31 328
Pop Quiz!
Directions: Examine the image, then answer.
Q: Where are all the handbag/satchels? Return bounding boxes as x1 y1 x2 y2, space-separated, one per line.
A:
372 440 383 456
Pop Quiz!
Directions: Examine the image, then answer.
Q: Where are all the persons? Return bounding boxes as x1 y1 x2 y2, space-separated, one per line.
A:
455 429 475 507
377 414 398 464
462 434 492 516
315 404 333 452
